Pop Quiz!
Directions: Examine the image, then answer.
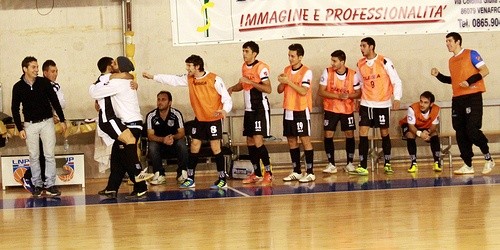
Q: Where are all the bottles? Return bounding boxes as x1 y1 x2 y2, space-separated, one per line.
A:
64 140 69 153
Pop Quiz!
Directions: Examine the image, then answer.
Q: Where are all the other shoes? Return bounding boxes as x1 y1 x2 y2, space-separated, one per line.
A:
135 167 154 183
98 188 117 199
209 179 227 189
481 158 495 174
32 187 43 196
383 163 394 175
47 186 62 196
255 172 274 187
20 177 34 194
433 161 443 172
177 170 187 183
124 190 150 199
242 172 262 184
148 171 166 185
179 178 196 189
299 173 316 183
283 172 303 181
453 165 474 174
408 164 418 173
344 162 355 172
349 167 369 176
322 163 337 173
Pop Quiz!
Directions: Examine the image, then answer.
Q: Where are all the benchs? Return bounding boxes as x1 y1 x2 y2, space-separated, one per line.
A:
141 119 500 181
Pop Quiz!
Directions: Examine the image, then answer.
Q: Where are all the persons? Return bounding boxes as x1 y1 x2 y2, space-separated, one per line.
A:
399 90 447 172
11 58 66 198
352 37 403 175
141 54 232 190
144 90 192 186
224 42 274 185
276 43 316 185
431 32 496 175
318 50 363 174
90 55 153 198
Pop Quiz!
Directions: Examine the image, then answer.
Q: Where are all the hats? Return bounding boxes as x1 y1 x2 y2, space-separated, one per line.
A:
117 56 134 73
